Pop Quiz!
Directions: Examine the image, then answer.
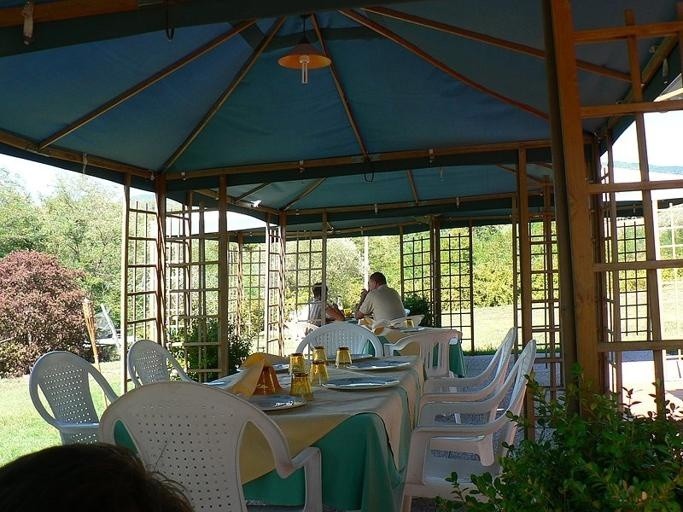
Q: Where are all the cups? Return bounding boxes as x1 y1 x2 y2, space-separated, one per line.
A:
344 309 352 318
406 319 414 327
288 353 306 375
312 345 329 366
308 360 328 385
335 346 353 370
289 372 313 401
352 313 355 317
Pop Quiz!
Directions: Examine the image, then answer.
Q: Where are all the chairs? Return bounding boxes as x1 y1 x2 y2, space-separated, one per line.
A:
390 313 426 327
399 338 538 511
97 381 324 512
126 339 192 387
296 321 320 340
295 321 384 357
29 350 119 446
384 328 461 425
420 326 518 468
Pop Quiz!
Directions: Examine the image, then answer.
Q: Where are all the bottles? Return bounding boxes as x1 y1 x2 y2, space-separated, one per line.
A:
337 295 343 311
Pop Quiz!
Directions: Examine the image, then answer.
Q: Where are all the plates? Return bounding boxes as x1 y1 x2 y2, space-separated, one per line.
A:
328 354 373 362
345 359 411 370
202 380 226 386
250 395 308 411
236 363 289 372
321 376 400 390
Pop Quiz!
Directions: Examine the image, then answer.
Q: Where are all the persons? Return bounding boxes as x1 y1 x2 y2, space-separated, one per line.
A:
305 283 345 337
355 272 406 325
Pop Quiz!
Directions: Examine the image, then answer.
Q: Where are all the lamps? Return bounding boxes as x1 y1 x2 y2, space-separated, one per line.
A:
276 37 336 85
